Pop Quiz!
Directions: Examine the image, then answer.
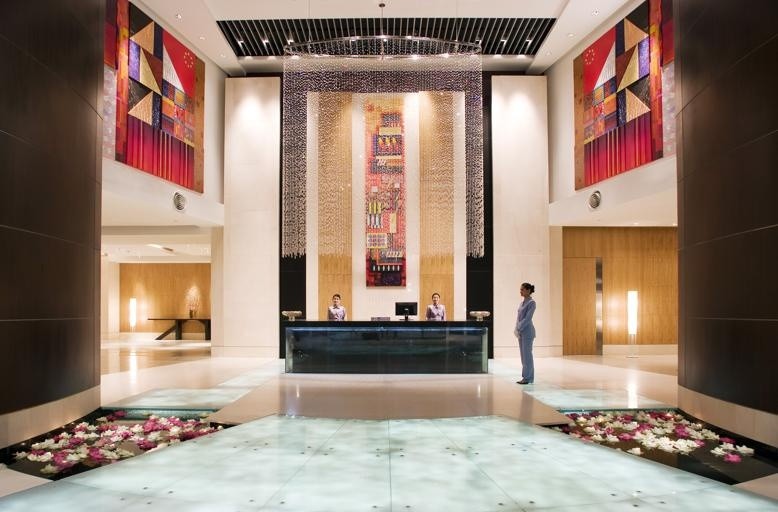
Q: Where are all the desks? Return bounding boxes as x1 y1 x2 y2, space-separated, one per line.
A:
147 318 211 340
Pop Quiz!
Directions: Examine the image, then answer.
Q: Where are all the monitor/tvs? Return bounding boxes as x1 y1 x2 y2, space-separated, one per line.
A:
395 302 418 320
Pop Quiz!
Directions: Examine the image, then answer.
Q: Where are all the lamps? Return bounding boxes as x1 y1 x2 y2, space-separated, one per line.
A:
278 3 485 262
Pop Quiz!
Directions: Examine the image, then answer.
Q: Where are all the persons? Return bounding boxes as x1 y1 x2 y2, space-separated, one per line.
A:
327 294 347 321
514 283 536 384
425 293 446 321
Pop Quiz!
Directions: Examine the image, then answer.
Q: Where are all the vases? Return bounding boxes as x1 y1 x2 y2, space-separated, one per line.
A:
189 310 194 319
